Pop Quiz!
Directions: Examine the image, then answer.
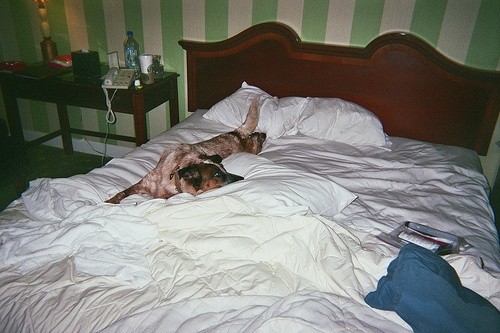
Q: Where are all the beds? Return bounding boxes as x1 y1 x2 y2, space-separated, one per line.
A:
0 21 500 333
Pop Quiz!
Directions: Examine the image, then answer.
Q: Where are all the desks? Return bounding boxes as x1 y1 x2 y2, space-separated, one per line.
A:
0 60 181 171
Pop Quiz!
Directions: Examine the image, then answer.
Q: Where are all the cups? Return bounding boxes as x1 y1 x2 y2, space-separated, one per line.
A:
138 53 153 73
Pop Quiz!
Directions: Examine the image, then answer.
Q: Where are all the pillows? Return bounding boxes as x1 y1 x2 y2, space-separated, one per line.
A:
202 81 312 140
167 150 359 217
286 97 393 152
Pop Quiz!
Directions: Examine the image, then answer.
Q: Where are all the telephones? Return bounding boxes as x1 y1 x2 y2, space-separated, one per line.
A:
102 67 135 89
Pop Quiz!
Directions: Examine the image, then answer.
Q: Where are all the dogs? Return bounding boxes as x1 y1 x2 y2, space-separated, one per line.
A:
101 92 269 207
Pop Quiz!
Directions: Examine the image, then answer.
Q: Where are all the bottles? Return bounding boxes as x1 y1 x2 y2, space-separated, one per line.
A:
124 30 142 78
133 69 144 90
147 55 164 80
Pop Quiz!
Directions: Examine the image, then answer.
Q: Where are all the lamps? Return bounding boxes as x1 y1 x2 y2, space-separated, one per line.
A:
33 0 59 61
